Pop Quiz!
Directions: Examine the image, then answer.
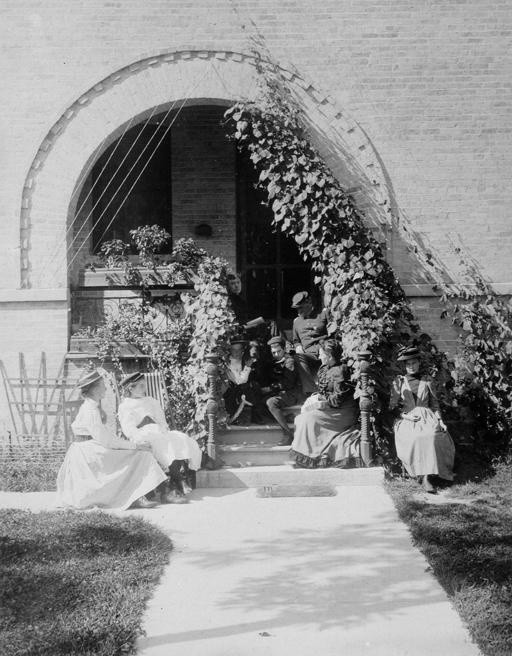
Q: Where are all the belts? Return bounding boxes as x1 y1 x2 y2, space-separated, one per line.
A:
136 415 156 429
73 433 93 443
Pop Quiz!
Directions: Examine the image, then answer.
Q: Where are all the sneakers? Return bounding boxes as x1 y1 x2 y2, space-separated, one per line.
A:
135 496 159 509
278 436 292 446
422 481 436 493
161 493 188 504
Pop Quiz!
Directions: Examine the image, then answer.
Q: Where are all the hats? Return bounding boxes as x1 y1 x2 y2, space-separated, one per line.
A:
291 291 309 308
78 371 103 389
119 371 145 388
267 336 286 346
319 339 343 357
397 348 426 362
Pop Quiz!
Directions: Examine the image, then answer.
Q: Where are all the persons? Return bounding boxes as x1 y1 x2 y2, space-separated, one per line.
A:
226 274 288 339
56 369 167 510
118 370 203 499
387 346 457 494
225 290 359 468
